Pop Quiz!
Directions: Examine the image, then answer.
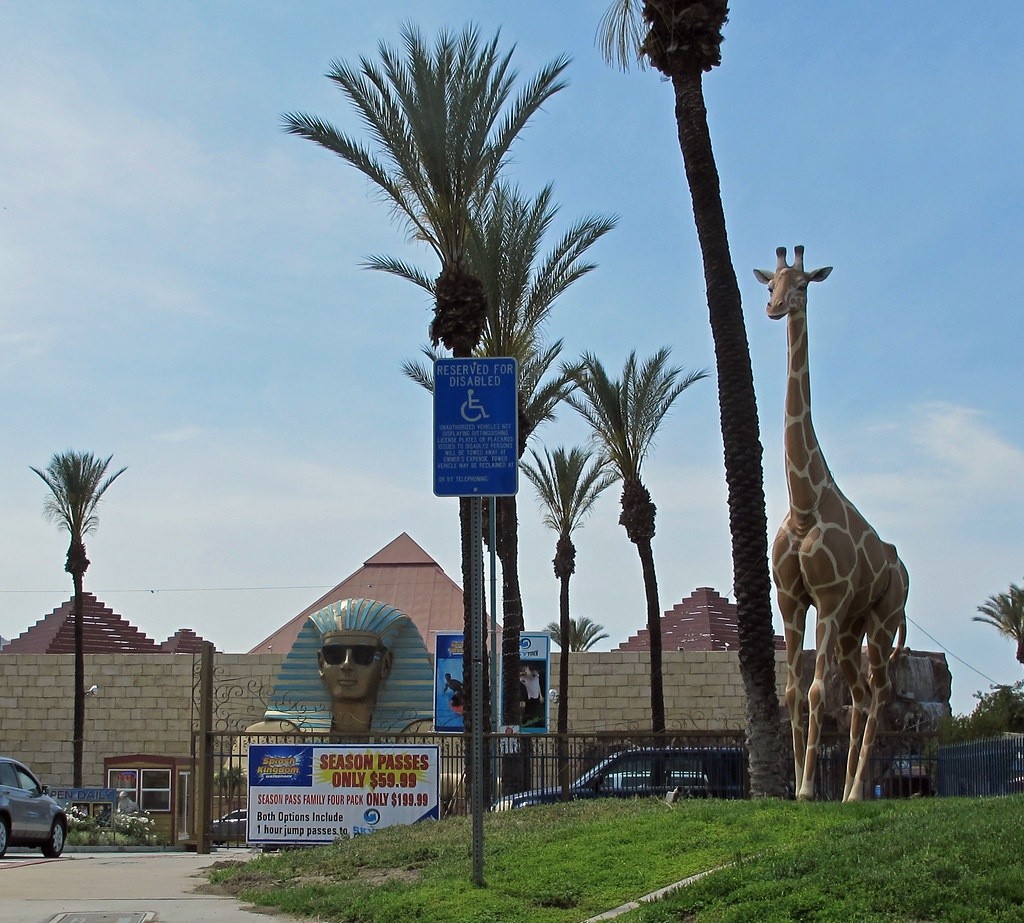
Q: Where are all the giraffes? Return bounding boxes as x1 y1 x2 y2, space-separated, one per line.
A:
751 244 908 800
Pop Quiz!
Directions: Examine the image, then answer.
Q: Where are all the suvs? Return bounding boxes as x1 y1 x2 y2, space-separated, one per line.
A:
491 745 795 812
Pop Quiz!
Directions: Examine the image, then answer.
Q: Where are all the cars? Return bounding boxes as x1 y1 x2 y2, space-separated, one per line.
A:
211 809 247 845
602 770 710 792
0 756 69 858
